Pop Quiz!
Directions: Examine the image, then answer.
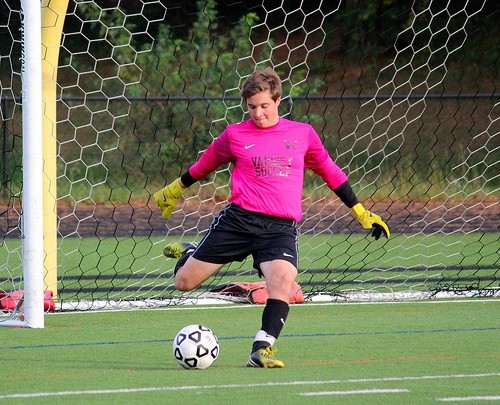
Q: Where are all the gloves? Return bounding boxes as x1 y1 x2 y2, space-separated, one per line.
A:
351 203 392 244
153 178 184 217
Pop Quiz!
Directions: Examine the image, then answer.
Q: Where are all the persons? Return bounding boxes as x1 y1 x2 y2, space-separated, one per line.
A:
154 68 390 368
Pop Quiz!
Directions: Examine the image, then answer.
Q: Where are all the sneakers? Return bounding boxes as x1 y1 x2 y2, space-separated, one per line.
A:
246 349 284 368
162 241 202 259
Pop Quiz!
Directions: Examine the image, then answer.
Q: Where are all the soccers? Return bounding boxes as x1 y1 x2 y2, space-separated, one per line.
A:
171 322 220 368
